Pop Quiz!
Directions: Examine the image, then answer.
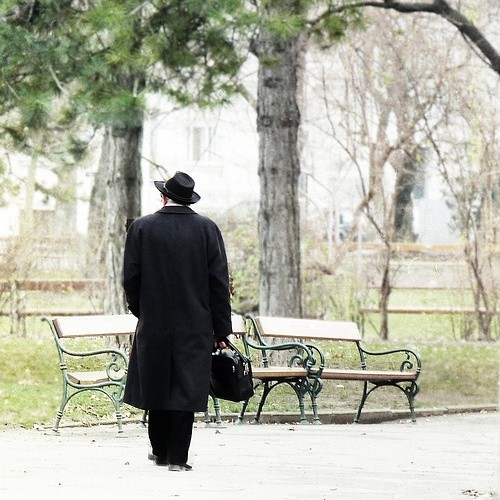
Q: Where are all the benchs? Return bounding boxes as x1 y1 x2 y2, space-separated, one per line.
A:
40 308 422 438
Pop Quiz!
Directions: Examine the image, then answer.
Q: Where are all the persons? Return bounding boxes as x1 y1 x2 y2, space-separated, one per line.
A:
122 172 232 471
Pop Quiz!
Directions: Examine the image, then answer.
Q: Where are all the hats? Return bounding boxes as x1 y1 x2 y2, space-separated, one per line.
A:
153 173 200 204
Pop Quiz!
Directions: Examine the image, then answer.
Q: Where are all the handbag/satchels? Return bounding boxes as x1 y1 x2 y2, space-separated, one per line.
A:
209 336 254 402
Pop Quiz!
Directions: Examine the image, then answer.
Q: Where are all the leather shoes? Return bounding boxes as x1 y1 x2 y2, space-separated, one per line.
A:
168 463 192 471
149 452 169 466
